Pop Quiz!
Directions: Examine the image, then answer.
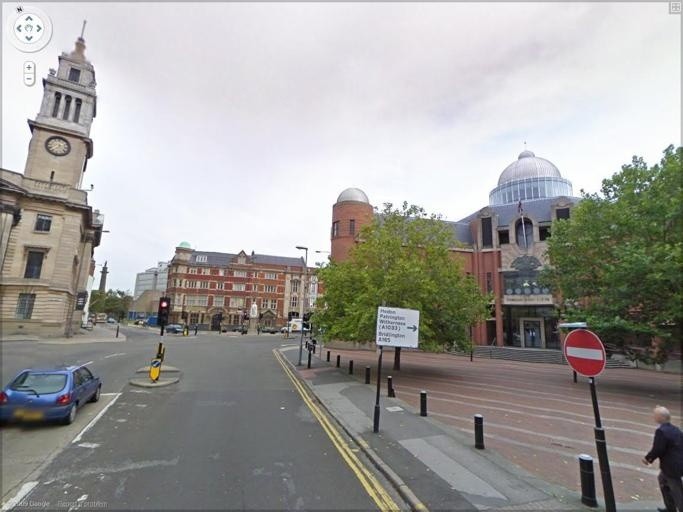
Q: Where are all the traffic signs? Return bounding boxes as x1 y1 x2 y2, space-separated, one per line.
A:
375 306 420 350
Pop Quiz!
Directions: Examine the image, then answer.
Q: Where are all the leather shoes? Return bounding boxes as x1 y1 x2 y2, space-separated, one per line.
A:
657 507 677 512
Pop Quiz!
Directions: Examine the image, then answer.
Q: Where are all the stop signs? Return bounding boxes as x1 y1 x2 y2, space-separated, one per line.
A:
563 329 606 378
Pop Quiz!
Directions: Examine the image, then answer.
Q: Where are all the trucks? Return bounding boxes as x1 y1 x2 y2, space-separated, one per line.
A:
280 319 310 332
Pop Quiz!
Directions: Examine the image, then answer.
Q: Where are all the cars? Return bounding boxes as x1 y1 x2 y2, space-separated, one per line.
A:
231 325 279 334
84 312 116 331
0 362 102 426
165 323 184 334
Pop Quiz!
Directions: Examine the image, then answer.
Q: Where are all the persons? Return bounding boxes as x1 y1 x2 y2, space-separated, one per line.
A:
642 406 683 512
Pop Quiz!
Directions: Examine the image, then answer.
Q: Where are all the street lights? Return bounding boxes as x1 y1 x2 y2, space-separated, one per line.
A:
315 249 331 254
295 246 308 368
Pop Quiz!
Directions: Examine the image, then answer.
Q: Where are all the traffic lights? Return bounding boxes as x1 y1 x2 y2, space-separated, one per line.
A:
288 312 292 321
303 313 309 328
156 297 170 326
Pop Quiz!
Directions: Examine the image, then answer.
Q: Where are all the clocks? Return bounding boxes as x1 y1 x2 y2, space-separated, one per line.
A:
45 136 71 155
